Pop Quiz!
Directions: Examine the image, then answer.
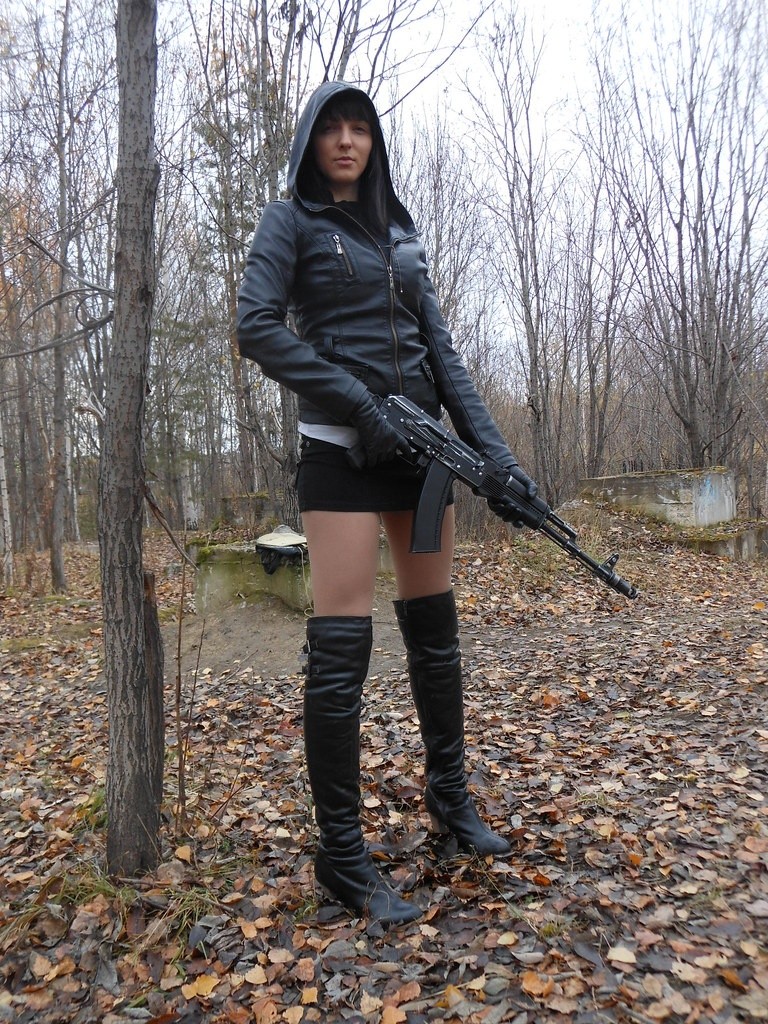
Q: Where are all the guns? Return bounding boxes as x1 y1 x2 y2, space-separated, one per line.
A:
341 392 640 602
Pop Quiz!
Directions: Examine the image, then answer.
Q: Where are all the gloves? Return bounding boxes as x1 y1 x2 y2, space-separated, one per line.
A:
488 465 538 529
348 390 412 474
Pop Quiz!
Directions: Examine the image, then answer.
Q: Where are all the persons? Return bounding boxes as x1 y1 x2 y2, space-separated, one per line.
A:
228 81 538 925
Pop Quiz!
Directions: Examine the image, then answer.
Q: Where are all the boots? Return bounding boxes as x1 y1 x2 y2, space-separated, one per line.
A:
302 615 425 931
393 587 512 858
